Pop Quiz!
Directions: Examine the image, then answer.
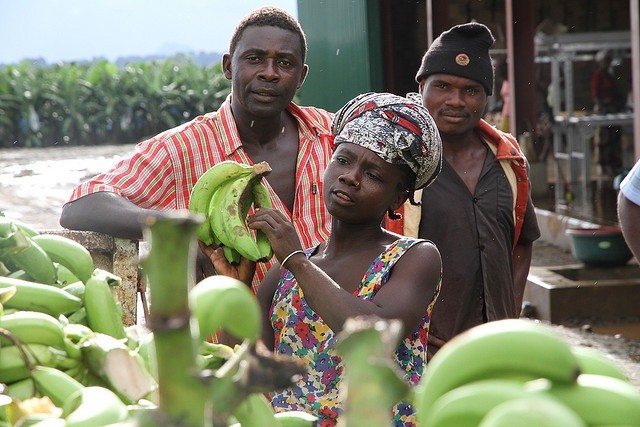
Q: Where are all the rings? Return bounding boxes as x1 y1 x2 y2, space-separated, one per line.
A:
214 247 223 253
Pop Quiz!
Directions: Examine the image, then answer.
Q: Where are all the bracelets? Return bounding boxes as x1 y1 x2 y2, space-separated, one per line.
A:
280 249 307 281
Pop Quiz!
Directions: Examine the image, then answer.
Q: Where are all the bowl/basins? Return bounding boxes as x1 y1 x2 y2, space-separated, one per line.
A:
564 227 636 265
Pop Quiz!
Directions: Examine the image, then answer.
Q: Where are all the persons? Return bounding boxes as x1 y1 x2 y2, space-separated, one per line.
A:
198 92 443 426
382 24 542 363
59 6 342 402
615 158 640 263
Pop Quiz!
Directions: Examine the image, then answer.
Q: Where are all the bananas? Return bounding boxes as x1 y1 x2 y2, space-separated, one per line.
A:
151 274 640 427
1 217 150 427
187 161 279 265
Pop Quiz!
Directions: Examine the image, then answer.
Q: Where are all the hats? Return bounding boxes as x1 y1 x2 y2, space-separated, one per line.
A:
416 22 497 97
